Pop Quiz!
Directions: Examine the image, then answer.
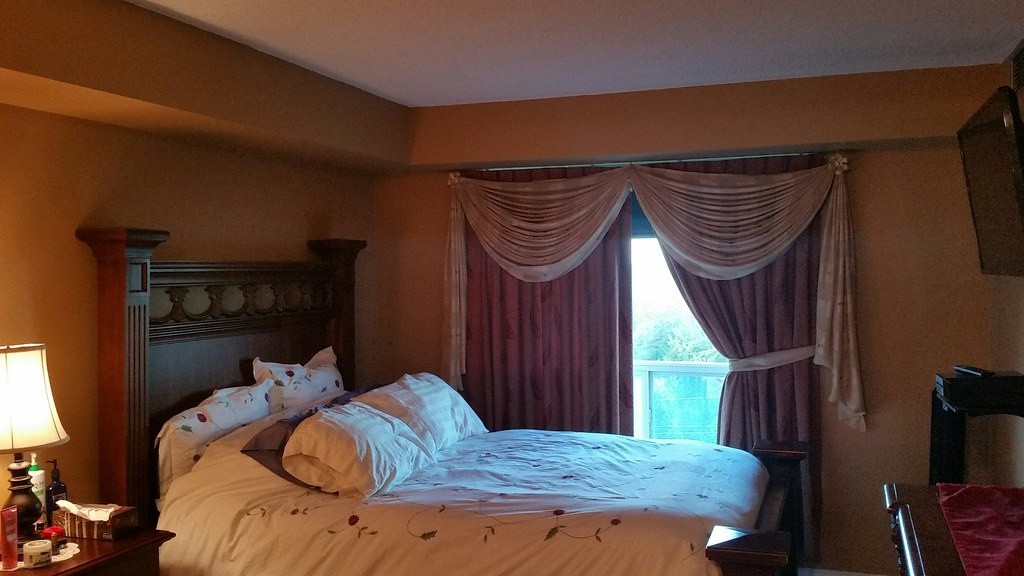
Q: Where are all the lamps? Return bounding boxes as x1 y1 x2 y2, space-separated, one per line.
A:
0 344 70 546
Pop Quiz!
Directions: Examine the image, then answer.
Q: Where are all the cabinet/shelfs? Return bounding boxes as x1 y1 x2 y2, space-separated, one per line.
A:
882 483 1024 576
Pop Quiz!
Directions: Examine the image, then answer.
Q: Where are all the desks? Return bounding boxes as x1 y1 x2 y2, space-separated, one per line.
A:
928 389 1024 484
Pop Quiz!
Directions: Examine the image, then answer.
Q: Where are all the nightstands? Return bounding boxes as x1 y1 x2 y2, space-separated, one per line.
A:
0 526 178 576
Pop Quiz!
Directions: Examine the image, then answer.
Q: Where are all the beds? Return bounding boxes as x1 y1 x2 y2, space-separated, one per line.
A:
154 427 810 576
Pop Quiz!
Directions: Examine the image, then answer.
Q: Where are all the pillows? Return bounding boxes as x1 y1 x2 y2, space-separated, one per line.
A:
240 371 489 501
254 346 343 416
155 378 275 500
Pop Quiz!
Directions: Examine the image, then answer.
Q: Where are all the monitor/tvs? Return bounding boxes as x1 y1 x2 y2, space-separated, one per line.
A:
956 84 1024 276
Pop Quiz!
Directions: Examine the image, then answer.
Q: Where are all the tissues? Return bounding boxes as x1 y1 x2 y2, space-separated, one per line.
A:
52 499 140 540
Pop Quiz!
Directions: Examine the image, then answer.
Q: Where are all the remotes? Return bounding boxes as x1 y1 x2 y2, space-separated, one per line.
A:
953 365 996 377
